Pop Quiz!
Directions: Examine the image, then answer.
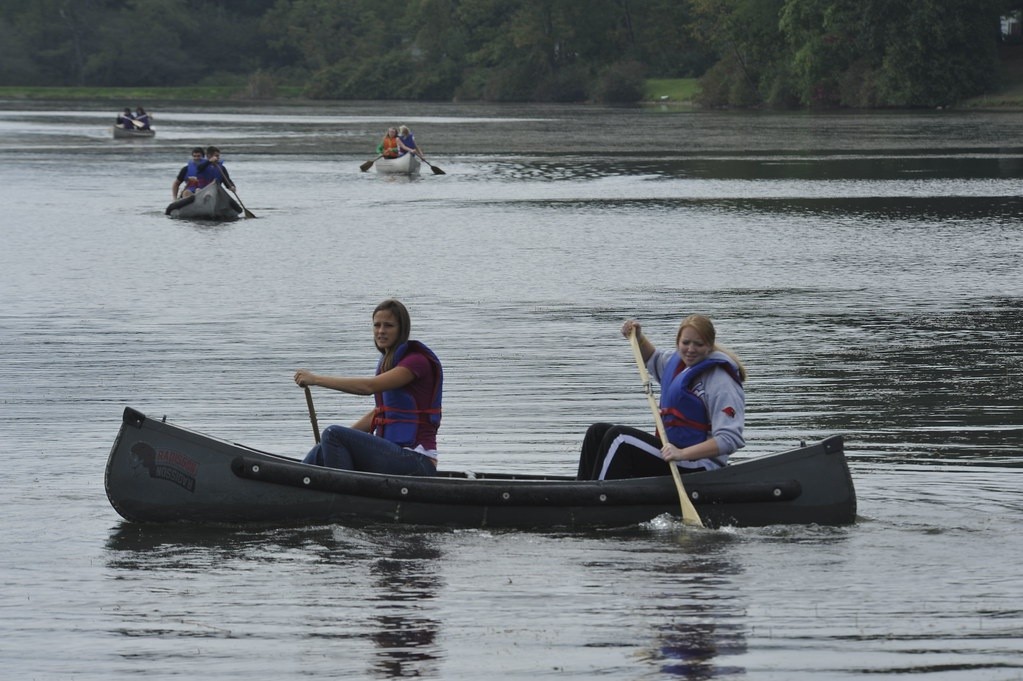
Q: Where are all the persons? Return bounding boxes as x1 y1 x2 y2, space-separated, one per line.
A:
378 127 416 159
293 301 445 477
171 147 237 203
397 126 423 156
117 107 150 131
577 315 745 481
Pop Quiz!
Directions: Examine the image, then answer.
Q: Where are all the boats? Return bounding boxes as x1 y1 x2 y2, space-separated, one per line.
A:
163 176 242 223
372 151 419 176
112 123 155 139
102 406 858 526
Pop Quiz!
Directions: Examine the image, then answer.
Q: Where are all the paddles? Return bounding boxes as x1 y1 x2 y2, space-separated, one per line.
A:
629 326 705 531
215 162 256 218
360 154 388 172
412 151 445 174
302 382 321 447
